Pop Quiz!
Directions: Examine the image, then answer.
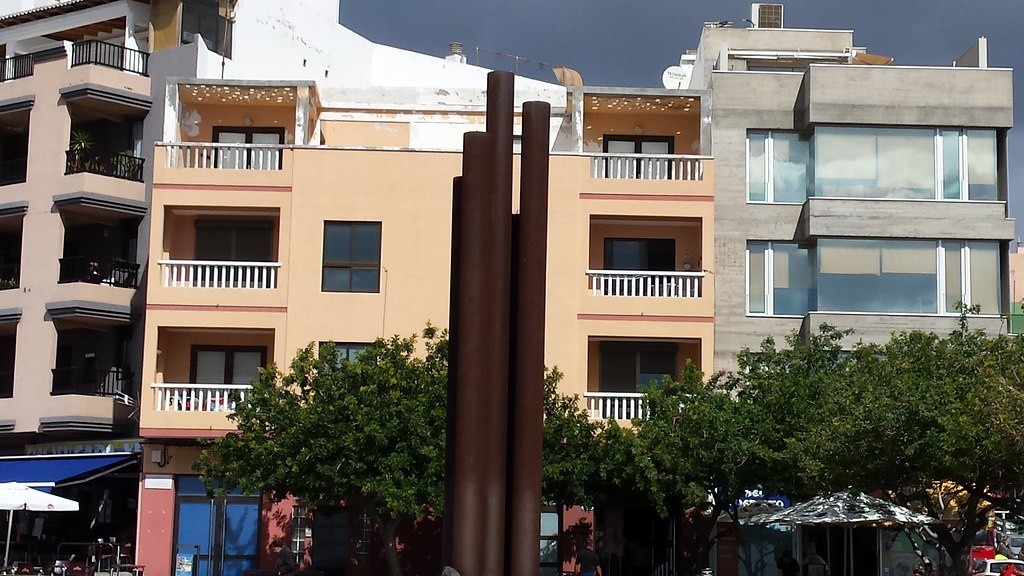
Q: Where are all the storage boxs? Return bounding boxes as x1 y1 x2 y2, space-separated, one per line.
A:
204 398 230 412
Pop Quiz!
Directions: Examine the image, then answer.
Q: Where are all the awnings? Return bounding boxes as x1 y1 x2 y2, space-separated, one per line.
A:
0 451 139 493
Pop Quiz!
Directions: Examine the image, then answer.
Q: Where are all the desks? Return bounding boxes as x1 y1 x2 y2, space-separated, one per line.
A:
111 564 146 576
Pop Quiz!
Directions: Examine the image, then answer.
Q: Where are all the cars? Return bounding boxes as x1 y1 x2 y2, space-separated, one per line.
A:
970 530 1024 576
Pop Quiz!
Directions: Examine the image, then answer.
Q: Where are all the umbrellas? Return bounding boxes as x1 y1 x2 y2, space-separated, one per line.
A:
708 480 995 576
0 482 79 568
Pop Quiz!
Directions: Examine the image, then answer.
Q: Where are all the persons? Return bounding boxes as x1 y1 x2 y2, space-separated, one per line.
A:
269 539 323 576
88 260 122 282
777 550 800 576
32 513 46 544
16 511 30 545
573 539 603 575
